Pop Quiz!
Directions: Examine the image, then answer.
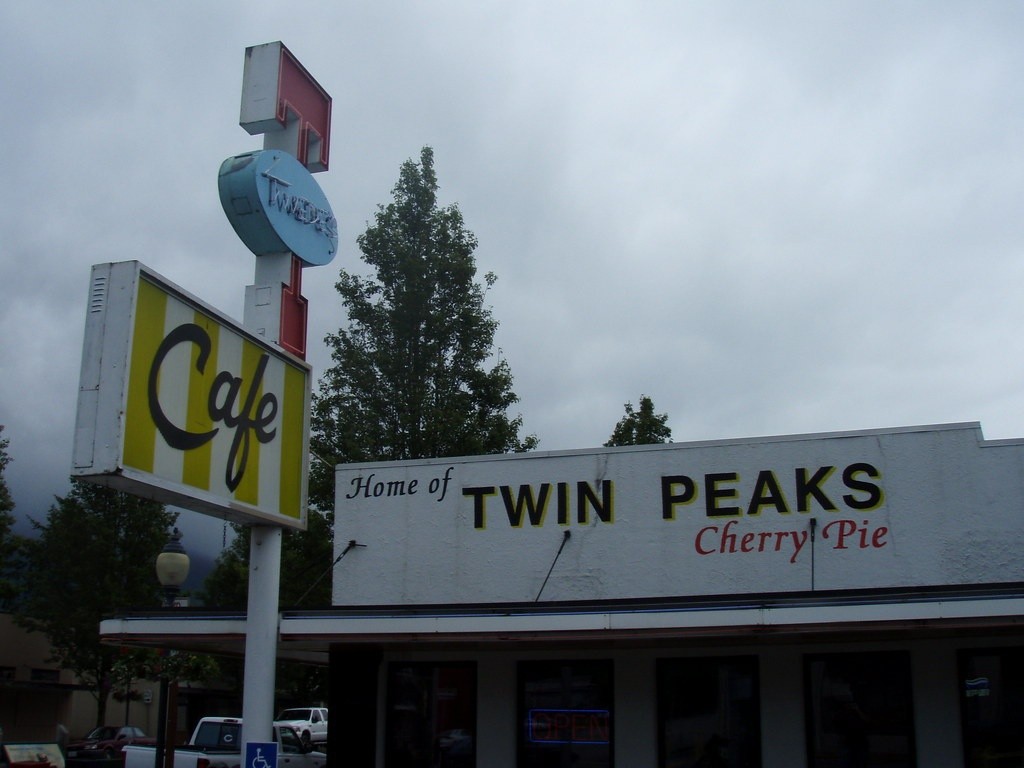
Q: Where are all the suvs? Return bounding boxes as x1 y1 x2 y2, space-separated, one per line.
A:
271 708 328 746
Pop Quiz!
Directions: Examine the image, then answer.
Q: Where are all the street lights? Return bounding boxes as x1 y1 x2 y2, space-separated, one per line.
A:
151 526 192 768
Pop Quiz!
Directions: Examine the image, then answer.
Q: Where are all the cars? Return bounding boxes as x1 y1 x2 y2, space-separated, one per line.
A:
60 725 157 768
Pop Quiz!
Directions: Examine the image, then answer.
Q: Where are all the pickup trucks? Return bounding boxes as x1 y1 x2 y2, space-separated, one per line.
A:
121 716 327 768
